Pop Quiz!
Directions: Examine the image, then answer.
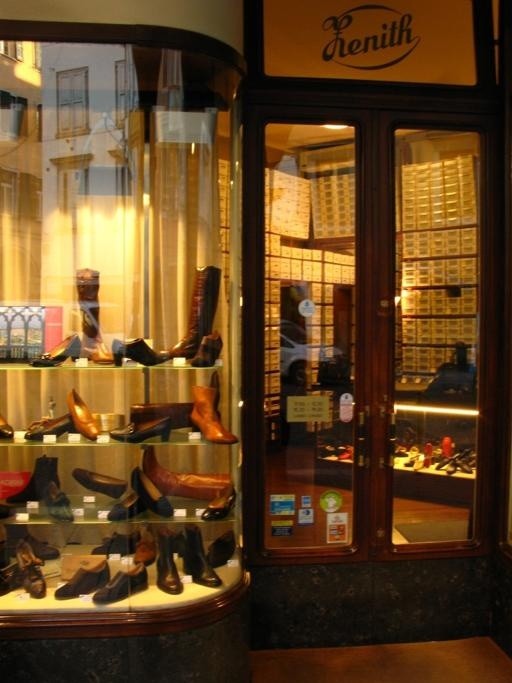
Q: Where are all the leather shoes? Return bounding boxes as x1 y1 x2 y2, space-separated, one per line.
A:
0 387 238 603
320 436 478 475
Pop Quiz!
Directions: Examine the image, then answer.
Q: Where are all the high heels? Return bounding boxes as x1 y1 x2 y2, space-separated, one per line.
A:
29 334 81 367
191 332 225 368
113 338 170 366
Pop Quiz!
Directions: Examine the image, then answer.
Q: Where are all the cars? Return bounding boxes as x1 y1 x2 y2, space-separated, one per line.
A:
279 320 344 385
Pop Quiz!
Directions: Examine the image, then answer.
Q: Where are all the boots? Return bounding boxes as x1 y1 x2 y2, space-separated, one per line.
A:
169 265 221 356
76 268 113 364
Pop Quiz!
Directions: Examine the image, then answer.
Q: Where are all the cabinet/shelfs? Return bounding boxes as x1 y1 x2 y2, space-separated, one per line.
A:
0 349 240 610
315 384 480 506
